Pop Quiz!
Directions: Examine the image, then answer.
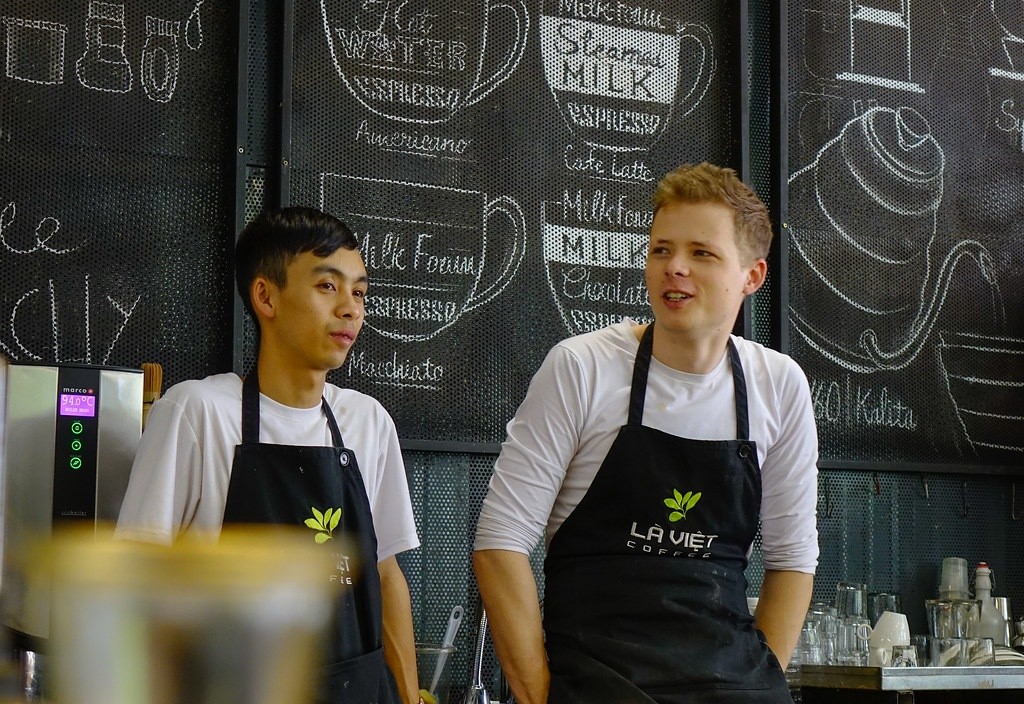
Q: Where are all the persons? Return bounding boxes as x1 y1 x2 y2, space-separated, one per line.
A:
112 205 423 704
472 161 820 704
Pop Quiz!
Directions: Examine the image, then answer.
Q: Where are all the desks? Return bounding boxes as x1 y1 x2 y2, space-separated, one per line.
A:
784 663 1024 704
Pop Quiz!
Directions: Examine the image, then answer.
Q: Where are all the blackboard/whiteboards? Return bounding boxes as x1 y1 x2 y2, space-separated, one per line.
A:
0 0 249 400
281 0 753 454
773 0 1024 474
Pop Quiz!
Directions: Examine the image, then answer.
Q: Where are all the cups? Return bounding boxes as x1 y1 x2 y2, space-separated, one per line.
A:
415 642 457 704
926 558 996 666
795 582 927 668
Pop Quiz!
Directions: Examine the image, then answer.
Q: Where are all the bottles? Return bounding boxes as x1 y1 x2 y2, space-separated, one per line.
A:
969 561 1005 645
